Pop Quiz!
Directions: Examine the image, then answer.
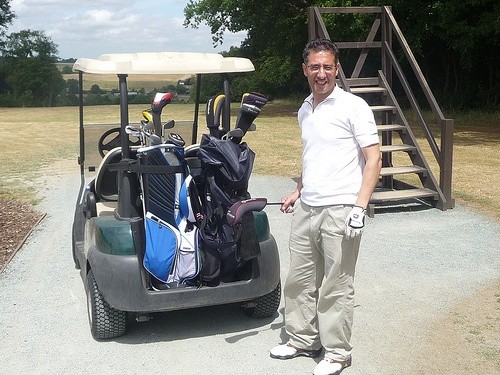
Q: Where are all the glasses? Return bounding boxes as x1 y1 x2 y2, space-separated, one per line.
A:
307 65 336 71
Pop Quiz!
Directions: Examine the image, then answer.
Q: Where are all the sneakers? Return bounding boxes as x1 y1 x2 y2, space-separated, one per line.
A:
313 353 351 375
270 342 322 358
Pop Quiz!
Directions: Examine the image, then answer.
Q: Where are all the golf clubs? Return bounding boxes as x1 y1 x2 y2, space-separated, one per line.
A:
221 124 256 141
124 119 185 147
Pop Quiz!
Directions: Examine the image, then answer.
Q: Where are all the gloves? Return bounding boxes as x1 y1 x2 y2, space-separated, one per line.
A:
345 205 367 239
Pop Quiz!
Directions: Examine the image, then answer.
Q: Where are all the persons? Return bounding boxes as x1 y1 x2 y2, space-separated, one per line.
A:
268 36 384 375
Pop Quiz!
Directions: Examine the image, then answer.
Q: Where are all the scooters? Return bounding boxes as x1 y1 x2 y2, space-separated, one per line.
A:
69 49 282 341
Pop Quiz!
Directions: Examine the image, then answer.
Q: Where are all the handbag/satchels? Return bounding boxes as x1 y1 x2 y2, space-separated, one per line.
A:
172 219 200 285
142 210 180 284
